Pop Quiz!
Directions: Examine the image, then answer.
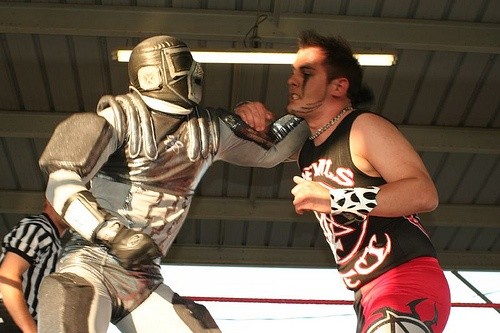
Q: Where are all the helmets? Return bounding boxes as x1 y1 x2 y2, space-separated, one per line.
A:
128 35 204 108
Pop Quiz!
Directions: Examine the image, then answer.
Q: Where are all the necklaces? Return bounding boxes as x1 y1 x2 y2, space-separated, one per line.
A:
309 104 350 142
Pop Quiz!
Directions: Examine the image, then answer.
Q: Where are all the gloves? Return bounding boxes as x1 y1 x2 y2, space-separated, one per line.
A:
107 226 162 271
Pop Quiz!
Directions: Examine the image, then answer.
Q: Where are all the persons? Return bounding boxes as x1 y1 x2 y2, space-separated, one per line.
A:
35 35 310 333
0 197 70 333
238 28 451 333
